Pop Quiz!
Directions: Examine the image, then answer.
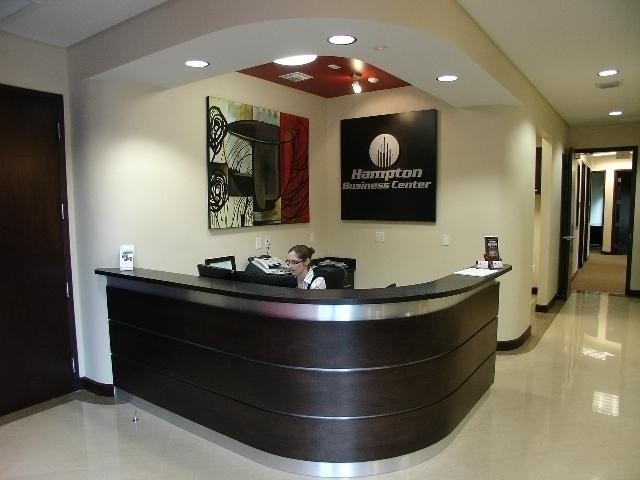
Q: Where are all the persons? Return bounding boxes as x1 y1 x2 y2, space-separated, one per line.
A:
284 244 326 290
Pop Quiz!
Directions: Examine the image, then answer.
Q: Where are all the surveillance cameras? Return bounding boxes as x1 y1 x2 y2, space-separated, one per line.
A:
573 153 581 161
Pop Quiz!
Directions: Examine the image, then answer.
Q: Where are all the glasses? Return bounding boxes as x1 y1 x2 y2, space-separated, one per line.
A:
285 260 305 265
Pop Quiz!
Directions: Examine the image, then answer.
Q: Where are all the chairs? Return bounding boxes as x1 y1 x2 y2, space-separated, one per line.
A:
314 264 347 289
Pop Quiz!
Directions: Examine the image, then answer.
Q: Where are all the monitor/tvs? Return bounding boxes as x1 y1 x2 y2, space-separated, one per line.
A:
204 255 236 270
197 262 236 283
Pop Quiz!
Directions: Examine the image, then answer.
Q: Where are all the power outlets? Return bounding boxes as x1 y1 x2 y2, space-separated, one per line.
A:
375 232 384 242
265 236 271 248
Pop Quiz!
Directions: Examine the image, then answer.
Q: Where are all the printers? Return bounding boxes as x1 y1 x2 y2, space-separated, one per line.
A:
244 254 298 288
